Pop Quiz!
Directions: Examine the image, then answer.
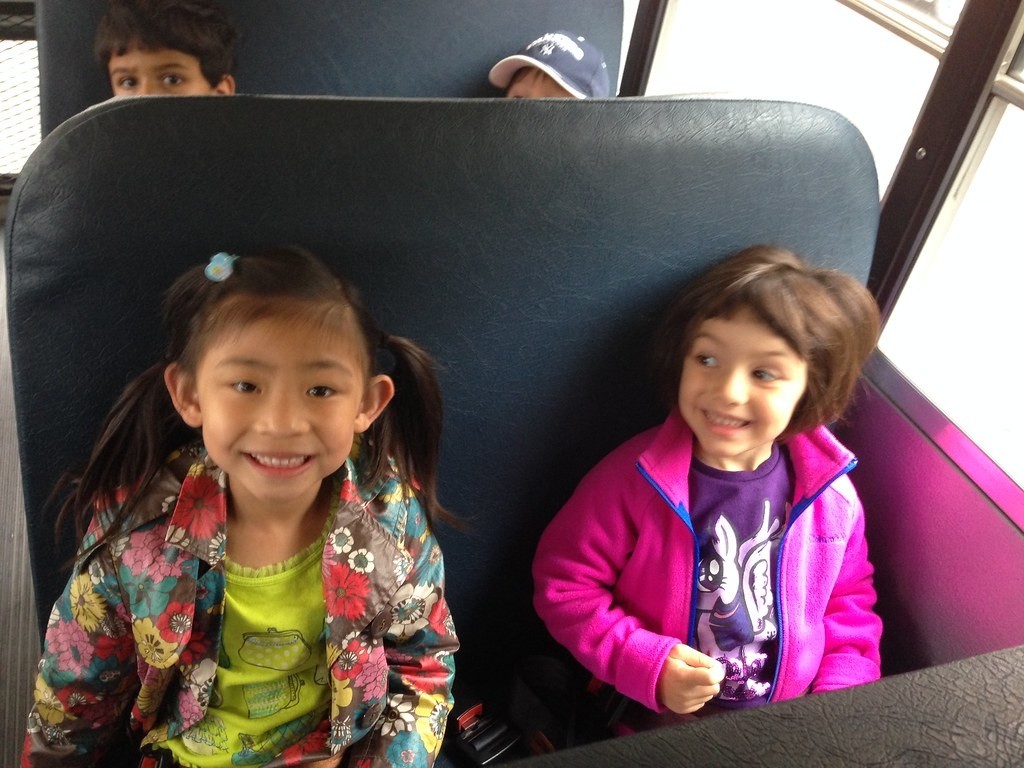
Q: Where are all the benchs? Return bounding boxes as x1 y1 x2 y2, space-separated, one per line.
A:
1 2 1024 768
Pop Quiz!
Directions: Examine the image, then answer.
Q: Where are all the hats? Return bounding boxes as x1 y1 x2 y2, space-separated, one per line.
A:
488 30 609 99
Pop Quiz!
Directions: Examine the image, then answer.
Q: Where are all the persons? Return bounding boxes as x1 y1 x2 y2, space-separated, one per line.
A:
488 30 610 98
92 0 236 97
21 245 460 768
532 244 883 747
